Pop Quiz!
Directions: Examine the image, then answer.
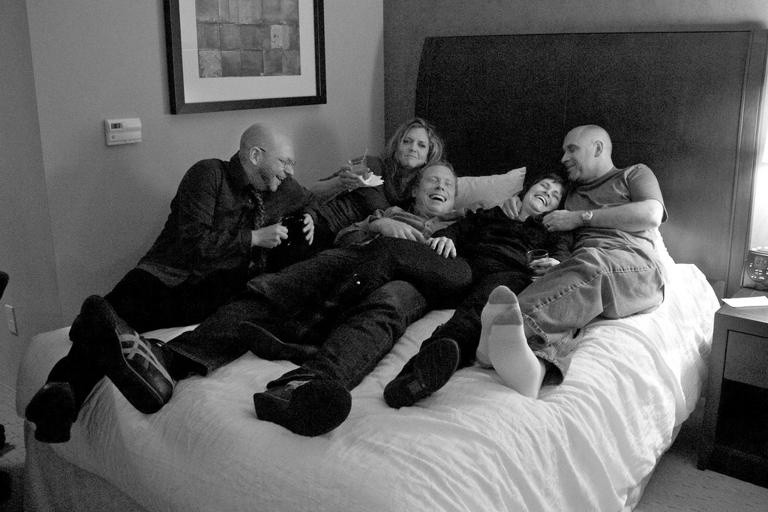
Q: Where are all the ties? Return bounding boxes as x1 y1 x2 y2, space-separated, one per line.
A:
247 188 267 284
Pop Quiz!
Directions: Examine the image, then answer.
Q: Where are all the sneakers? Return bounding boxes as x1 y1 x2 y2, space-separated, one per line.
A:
383 338 461 410
252 375 352 438
24 381 76 444
79 293 178 415
238 316 321 367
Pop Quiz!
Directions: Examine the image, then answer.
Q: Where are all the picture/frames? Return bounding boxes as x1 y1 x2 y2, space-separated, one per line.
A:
163 0 327 114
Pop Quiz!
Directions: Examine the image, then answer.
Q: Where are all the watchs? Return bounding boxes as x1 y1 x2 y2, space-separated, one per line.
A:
581 208 592 229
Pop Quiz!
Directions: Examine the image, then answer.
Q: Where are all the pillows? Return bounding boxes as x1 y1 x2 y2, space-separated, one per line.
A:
455 165 528 210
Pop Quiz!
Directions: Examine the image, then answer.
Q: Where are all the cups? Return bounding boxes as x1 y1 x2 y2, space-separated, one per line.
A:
346 153 369 180
282 213 306 247
526 249 550 282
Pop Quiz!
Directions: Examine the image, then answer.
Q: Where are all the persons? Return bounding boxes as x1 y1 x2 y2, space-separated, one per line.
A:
475 124 669 401
80 160 463 438
240 118 444 279
238 172 572 409
25 122 321 443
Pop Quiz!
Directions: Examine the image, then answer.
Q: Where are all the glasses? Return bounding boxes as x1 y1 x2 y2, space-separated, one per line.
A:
259 147 297 167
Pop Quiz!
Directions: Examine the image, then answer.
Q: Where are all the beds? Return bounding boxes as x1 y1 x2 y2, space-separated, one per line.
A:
16 24 768 512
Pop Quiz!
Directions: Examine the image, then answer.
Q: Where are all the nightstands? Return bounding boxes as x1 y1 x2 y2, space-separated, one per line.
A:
696 288 768 488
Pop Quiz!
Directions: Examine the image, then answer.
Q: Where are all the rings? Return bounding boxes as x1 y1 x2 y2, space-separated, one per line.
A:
546 223 551 228
